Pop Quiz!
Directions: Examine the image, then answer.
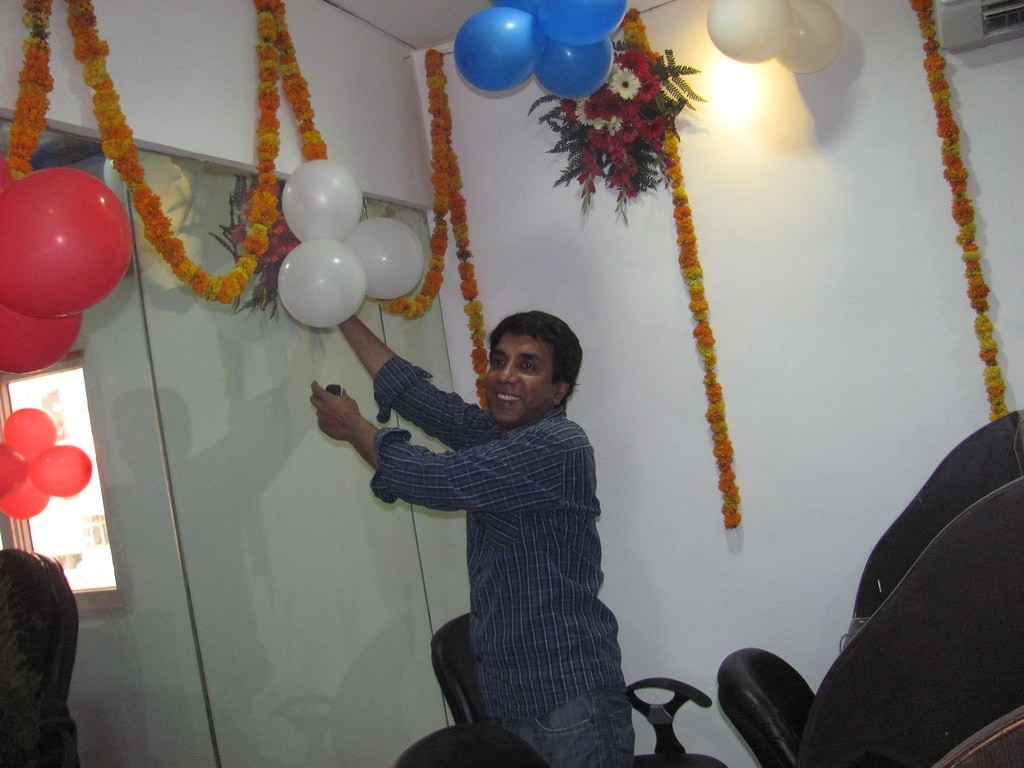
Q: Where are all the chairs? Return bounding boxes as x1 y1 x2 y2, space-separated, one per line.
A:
430 612 729 768
717 648 816 768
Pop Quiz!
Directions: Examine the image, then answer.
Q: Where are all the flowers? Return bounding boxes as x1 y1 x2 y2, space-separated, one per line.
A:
528 38 707 227
207 171 300 320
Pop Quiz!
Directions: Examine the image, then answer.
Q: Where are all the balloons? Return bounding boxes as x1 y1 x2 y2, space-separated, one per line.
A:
278 158 426 329
102 148 204 289
0 408 94 520
708 0 840 73
455 0 627 98
0 155 134 376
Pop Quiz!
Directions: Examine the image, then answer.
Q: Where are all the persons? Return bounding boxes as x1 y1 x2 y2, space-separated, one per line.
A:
311 310 634 767
